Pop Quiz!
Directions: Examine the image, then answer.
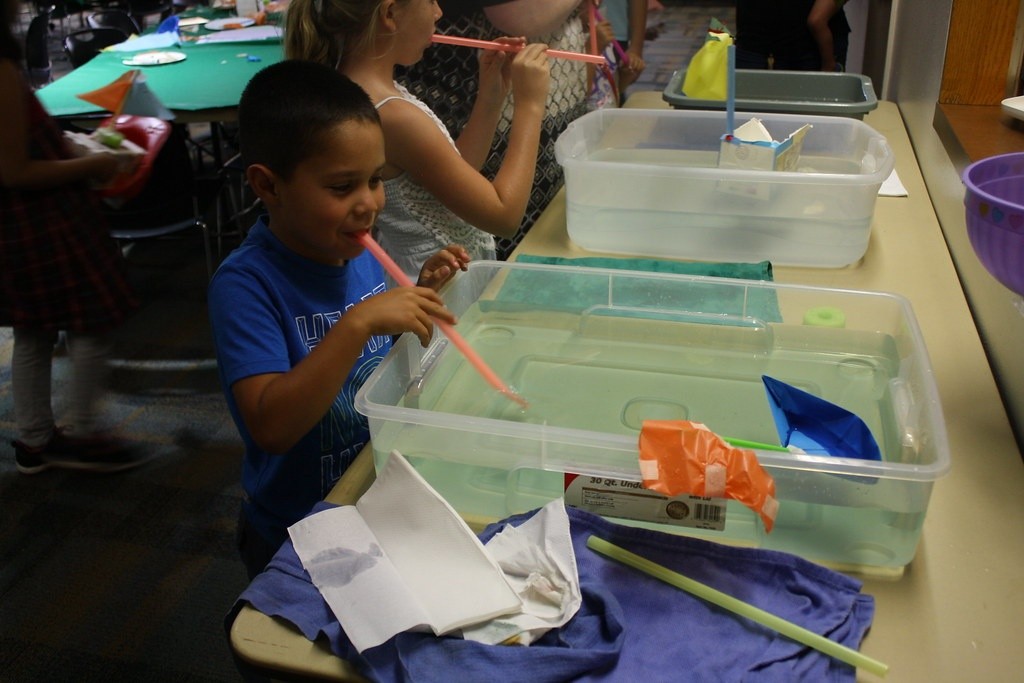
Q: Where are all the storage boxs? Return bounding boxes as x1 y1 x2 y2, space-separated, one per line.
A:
353 259 954 569
659 64 878 121
554 105 896 270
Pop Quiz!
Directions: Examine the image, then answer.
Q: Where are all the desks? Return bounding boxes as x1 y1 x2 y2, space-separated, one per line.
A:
227 98 1024 682
35 7 285 242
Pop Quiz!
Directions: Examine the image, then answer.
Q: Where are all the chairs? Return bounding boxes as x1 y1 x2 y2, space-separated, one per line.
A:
24 0 264 372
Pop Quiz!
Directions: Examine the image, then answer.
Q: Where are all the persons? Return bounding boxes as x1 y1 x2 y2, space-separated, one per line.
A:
206 61 470 583
284 0 553 284
391 0 598 262
736 0 850 71
0 0 153 475
582 0 645 114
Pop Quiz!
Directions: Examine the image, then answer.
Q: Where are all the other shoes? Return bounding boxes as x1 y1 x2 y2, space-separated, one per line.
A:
47 433 155 472
11 439 52 475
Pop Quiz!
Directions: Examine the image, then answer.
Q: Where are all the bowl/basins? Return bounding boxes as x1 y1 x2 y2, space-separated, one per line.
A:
960 152 1024 297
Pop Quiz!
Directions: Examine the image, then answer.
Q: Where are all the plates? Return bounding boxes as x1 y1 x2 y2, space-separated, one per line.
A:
1000 95 1024 122
